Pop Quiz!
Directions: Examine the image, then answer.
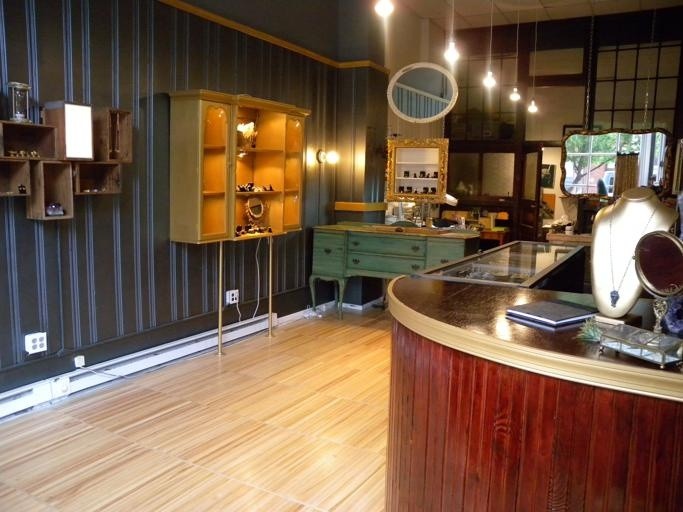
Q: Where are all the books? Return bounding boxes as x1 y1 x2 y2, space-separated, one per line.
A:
505 299 596 327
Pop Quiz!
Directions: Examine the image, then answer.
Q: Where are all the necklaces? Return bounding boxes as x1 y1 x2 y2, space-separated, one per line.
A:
607 201 656 307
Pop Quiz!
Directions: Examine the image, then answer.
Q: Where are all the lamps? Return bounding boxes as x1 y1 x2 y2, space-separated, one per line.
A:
443 0 539 113
316 149 339 163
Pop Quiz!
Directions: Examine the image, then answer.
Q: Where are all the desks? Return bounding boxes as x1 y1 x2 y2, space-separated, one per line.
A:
479 227 510 246
310 224 480 320
547 231 591 247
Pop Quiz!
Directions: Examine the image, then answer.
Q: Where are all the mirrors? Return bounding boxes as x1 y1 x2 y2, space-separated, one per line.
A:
560 128 671 199
387 62 459 123
631 230 683 335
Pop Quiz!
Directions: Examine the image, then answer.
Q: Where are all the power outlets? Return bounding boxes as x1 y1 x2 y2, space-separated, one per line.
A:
226 289 239 304
25 332 47 354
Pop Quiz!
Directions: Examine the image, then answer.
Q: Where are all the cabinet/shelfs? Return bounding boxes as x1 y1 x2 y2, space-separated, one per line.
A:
0 101 133 221
386 138 448 204
169 89 311 244
448 141 543 243
